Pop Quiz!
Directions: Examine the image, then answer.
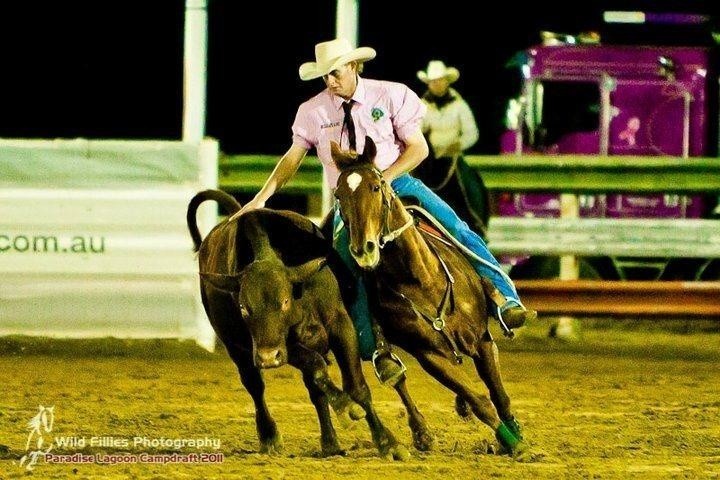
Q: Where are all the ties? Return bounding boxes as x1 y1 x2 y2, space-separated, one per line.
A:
338 101 356 150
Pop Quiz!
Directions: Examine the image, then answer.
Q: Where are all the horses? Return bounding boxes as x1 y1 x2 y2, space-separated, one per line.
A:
320 135 533 463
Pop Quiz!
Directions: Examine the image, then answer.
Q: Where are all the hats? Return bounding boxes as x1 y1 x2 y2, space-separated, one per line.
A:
298 37 378 82
416 59 461 86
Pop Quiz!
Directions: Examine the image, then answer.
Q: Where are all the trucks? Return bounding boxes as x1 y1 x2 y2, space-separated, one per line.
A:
467 9 719 283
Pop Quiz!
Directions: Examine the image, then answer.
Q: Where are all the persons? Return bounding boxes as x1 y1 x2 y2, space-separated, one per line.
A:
416 59 481 159
228 36 537 382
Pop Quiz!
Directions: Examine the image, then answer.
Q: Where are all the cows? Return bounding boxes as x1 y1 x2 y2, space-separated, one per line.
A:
185 188 413 464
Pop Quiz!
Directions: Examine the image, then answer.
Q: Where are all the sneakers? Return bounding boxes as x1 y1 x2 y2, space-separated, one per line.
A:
374 355 407 385
499 306 538 332
